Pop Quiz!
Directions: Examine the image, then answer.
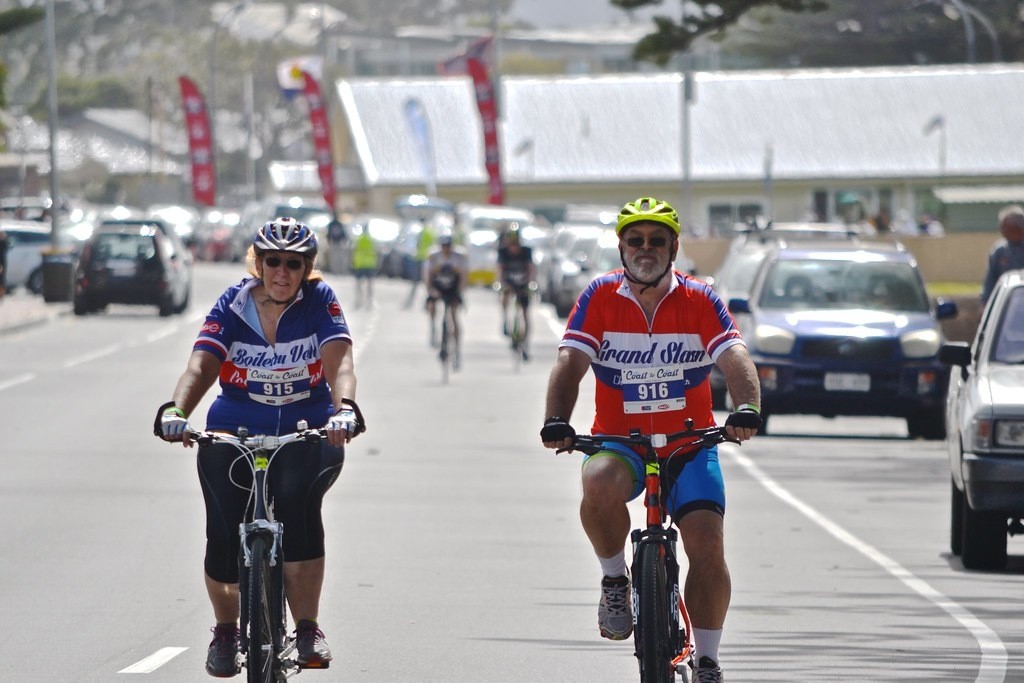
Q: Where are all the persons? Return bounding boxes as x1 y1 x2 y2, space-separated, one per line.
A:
154 215 355 677
326 217 345 272
351 223 375 304
425 235 465 346
540 198 761 683
982 206 1024 301
404 217 432 304
497 229 535 361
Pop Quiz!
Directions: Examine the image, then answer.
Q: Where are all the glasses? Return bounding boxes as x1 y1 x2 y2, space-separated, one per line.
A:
265 257 305 270
622 236 668 248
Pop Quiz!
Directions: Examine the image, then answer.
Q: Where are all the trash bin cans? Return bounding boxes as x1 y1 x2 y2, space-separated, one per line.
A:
42 253 75 303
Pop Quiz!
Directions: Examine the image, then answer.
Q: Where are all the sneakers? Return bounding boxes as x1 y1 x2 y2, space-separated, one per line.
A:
597 574 634 640
291 620 333 665
689 655 723 683
206 623 241 677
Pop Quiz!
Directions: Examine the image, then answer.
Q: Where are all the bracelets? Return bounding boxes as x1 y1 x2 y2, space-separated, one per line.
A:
736 403 760 415
164 408 184 417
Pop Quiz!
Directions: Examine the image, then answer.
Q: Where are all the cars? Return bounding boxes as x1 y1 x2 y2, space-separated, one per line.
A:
0 190 699 370
711 220 960 442
936 266 1024 575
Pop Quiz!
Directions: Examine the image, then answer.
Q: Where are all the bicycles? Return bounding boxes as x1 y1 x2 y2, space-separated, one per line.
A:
553 416 744 683
492 276 535 360
160 416 354 683
426 281 463 365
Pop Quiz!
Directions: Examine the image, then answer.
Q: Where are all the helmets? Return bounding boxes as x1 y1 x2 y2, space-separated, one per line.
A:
616 198 681 236
252 216 319 262
